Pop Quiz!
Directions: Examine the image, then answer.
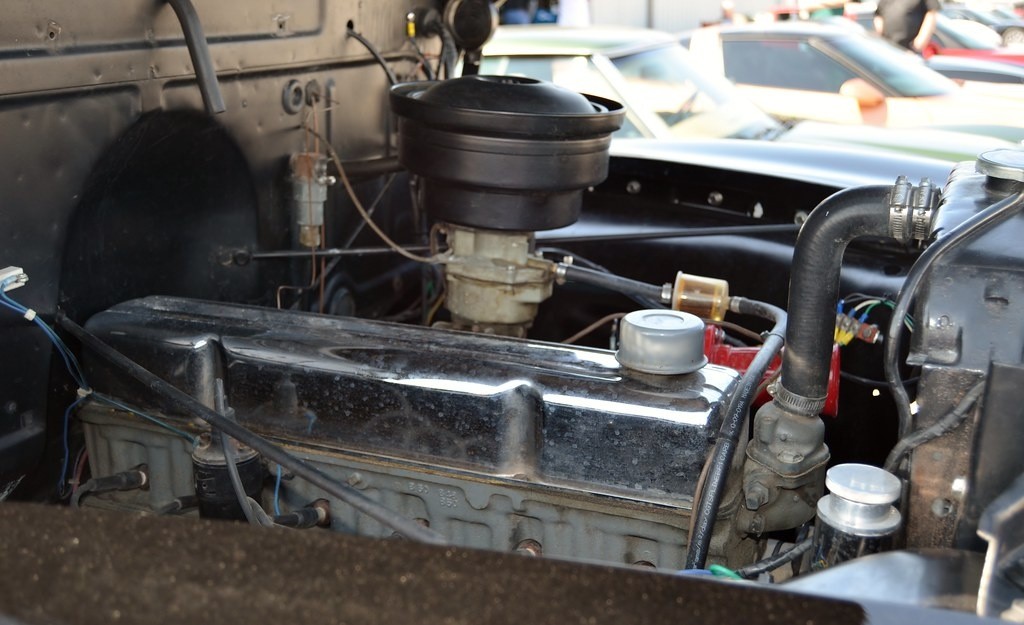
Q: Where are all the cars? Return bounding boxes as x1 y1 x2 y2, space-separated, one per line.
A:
452 0 1024 206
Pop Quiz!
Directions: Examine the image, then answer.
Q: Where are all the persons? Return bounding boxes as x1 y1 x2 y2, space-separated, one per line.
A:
872 0 942 56
796 0 844 21
720 1 735 23
